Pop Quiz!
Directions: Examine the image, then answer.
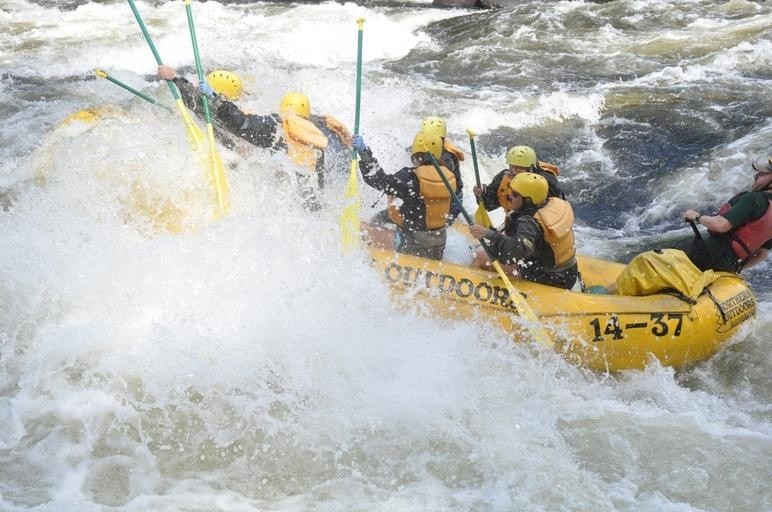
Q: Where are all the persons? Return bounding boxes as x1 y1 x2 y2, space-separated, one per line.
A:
370 115 465 234
186 75 327 226
583 152 772 301
473 143 568 275
156 61 246 150
310 116 355 177
350 131 458 263
467 170 588 295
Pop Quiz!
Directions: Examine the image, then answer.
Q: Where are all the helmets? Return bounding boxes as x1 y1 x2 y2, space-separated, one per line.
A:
279 92 310 121
506 145 538 170
207 71 242 102
419 116 447 136
410 131 442 166
509 172 550 206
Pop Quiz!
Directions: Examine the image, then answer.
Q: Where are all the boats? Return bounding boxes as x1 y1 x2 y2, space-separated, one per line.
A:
28 105 756 373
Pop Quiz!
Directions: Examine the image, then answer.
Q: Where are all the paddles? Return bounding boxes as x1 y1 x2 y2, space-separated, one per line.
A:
127 0 206 155
463 127 497 232
428 149 552 352
340 16 367 251
180 0 232 222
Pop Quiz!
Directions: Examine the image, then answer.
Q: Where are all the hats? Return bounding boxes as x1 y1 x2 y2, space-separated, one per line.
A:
752 159 772 173
